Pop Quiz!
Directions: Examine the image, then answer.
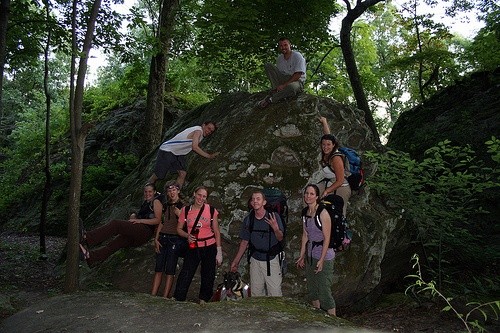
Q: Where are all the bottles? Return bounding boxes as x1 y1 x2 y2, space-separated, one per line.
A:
190 228 200 248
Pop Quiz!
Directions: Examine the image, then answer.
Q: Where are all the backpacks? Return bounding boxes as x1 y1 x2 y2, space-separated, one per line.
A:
302 194 343 265
319 147 363 194
150 191 166 211
248 189 288 276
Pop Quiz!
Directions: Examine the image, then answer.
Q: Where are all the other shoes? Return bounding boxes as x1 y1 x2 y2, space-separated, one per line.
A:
260 95 271 107
79 244 89 261
78 219 87 242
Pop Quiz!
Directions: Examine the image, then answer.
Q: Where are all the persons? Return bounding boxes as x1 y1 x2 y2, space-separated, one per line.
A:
295 184 336 317
231 192 283 297
171 186 223 304
257 37 306 108
151 181 185 297
149 121 220 192
315 117 351 218
80 184 162 269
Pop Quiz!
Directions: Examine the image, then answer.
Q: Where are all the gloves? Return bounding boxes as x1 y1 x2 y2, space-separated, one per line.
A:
216 247 222 265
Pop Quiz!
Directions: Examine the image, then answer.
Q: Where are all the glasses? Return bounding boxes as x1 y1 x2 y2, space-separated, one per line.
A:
320 144 332 146
169 186 179 189
